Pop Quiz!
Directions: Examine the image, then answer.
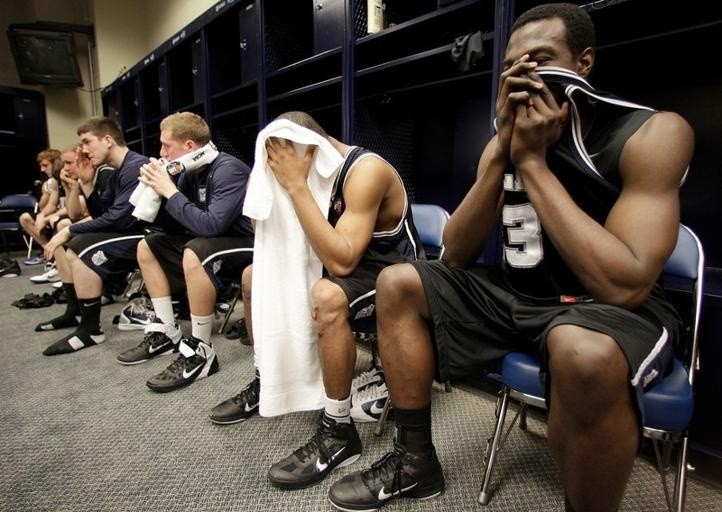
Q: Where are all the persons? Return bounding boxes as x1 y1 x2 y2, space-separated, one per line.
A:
325 2 695 512
18 109 253 394
207 111 427 489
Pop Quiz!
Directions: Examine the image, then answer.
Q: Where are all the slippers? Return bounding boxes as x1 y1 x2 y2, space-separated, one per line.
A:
225 318 252 345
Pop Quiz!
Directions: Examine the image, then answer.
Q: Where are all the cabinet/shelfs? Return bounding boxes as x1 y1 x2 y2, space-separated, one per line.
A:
349 1 500 393
502 0 719 482
102 20 206 161
207 0 261 169
261 0 347 145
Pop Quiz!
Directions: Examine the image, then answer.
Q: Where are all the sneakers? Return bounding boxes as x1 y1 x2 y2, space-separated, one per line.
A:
117 297 179 330
268 411 361 490
350 356 385 395
23 249 115 307
146 334 219 392
328 436 446 512
116 317 184 365
209 378 259 424
349 380 394 421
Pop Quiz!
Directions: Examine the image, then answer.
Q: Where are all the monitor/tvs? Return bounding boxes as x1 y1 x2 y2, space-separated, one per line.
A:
6 22 86 89
